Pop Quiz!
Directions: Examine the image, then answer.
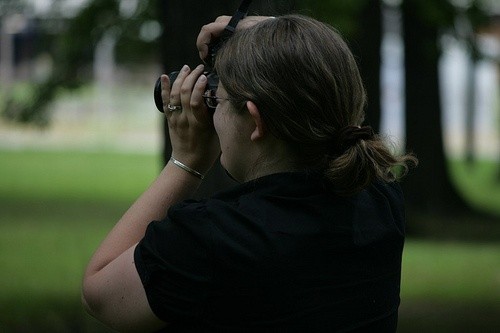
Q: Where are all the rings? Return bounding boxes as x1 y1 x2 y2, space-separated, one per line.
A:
167 104 181 111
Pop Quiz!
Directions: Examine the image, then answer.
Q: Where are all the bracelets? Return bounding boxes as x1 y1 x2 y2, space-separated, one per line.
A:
169 157 204 180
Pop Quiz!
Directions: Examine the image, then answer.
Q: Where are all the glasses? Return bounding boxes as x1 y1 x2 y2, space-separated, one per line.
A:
202 89 232 109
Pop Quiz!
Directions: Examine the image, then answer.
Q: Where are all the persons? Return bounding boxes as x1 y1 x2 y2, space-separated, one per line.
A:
81 14 404 333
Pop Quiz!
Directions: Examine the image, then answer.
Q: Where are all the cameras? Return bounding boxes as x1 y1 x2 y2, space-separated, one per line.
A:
154 37 225 117
